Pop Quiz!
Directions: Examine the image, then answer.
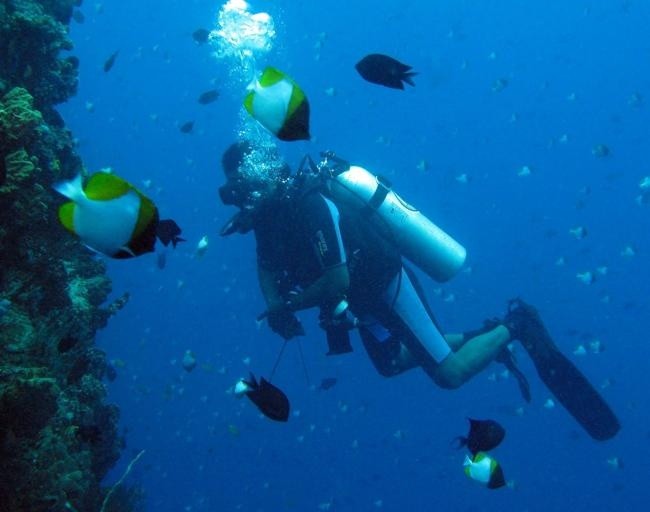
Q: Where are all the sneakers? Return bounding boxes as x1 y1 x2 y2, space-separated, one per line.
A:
507 305 559 371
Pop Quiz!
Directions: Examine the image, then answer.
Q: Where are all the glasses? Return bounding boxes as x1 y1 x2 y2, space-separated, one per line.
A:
220 176 267 204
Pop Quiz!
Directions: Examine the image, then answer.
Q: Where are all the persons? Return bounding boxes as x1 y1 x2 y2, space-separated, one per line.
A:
219 138 555 391
